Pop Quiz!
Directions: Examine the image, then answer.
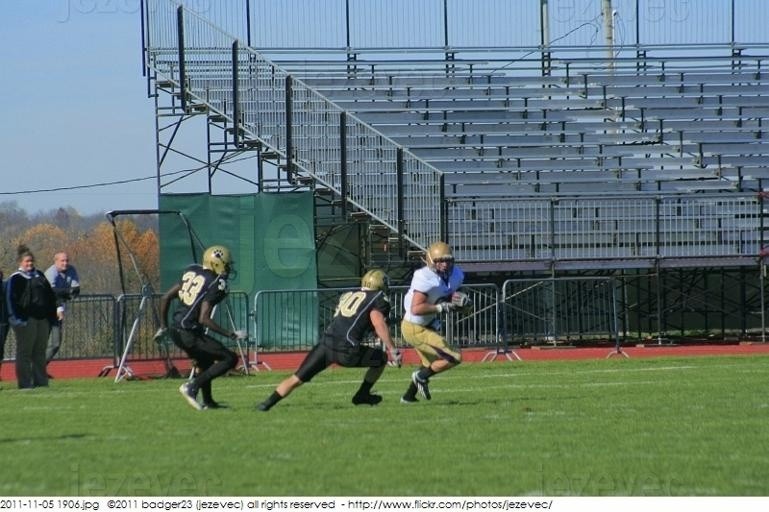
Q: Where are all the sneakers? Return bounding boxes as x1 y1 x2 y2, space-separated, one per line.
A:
180 382 203 410
353 394 381 404
411 371 431 400
201 402 228 410
255 403 267 411
401 395 418 404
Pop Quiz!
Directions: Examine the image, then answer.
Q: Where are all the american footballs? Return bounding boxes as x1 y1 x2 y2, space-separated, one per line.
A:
450 290 468 307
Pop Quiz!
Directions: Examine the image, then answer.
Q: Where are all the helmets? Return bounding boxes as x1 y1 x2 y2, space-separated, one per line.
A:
361 269 389 294
427 242 454 277
203 246 237 280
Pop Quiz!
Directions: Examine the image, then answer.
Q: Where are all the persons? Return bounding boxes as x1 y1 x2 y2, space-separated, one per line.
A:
149 245 251 411
0 268 13 368
6 244 56 389
256 269 402 411
400 241 473 403
41 250 82 379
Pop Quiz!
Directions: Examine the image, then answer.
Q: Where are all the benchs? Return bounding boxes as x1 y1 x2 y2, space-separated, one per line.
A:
154 38 743 257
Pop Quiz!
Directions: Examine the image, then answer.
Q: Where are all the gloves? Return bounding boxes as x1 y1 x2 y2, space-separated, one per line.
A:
388 349 402 367
231 331 247 340
153 327 168 341
438 301 456 312
